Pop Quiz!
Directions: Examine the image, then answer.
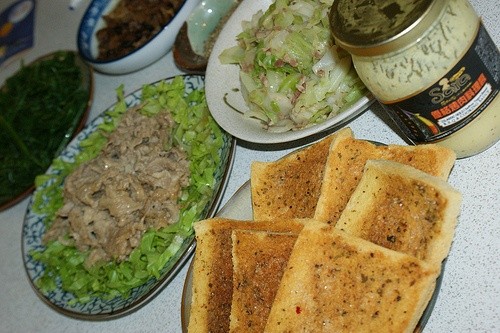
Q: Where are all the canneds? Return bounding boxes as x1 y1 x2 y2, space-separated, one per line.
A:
330 0 499 159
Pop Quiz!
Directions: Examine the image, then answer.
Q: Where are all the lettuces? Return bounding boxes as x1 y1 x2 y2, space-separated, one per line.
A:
30 76 223 306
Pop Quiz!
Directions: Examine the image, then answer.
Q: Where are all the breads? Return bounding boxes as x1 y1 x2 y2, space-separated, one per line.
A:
185 126 459 333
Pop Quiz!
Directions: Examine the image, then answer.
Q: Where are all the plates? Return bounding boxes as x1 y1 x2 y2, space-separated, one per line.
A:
19 73 237 320
0 48 95 213
171 1 237 75
202 0 377 144
73 0 202 74
175 137 443 333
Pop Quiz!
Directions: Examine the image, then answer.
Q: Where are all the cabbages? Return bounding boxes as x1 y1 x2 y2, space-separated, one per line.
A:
218 1 366 134
